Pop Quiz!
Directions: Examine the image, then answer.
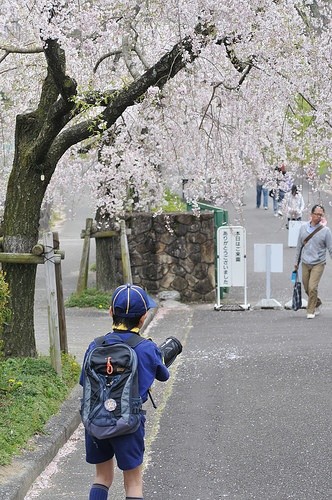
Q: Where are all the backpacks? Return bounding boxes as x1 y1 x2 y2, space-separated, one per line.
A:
79 334 147 439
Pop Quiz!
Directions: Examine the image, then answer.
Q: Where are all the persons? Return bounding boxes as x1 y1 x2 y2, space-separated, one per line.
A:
79 284 170 500
290 204 332 319
256 167 305 230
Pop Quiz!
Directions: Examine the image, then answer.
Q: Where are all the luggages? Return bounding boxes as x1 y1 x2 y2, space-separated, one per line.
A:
288 212 302 248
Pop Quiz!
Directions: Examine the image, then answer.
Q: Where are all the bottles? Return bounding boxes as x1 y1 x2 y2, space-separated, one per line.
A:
291 270 297 283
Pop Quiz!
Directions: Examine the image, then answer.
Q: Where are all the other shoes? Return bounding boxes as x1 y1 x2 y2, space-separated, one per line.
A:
278 211 283 216
315 304 322 315
256 206 259 208
307 314 315 319
264 207 268 209
274 212 278 216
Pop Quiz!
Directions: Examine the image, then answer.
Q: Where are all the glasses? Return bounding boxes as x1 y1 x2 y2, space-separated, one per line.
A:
313 213 322 216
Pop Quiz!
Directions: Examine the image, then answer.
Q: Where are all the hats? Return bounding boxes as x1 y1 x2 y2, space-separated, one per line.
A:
111 284 156 316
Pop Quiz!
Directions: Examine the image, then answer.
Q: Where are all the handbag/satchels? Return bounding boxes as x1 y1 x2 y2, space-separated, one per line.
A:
292 271 302 311
